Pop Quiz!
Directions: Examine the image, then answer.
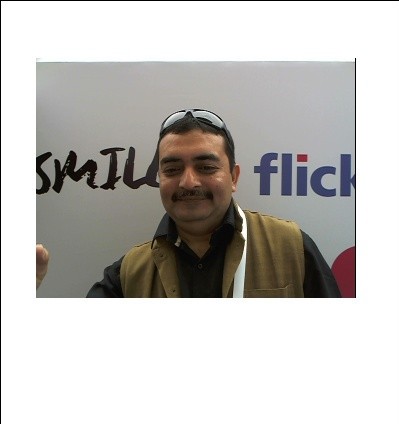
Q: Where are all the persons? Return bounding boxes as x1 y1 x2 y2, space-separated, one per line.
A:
36 108 340 298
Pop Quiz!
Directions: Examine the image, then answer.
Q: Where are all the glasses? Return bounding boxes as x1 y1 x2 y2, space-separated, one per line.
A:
159 109 234 151
